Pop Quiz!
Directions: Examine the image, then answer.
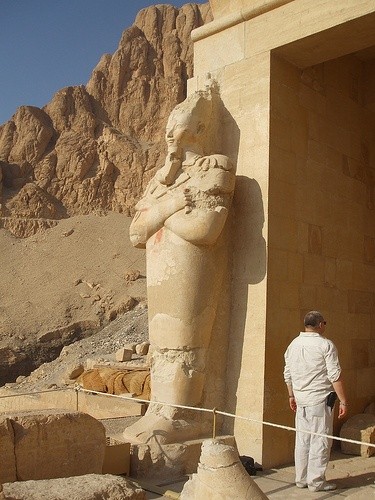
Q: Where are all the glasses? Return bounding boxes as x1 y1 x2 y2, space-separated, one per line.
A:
321 321 327 325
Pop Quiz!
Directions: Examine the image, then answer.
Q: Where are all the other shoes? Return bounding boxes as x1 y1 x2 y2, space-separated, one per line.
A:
295 483 307 488
320 482 338 490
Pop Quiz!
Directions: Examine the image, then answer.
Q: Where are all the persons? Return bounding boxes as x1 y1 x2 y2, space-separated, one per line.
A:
283 311 349 492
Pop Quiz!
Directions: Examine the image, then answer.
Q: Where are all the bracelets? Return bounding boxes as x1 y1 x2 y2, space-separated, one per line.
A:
288 396 294 398
340 402 348 406
